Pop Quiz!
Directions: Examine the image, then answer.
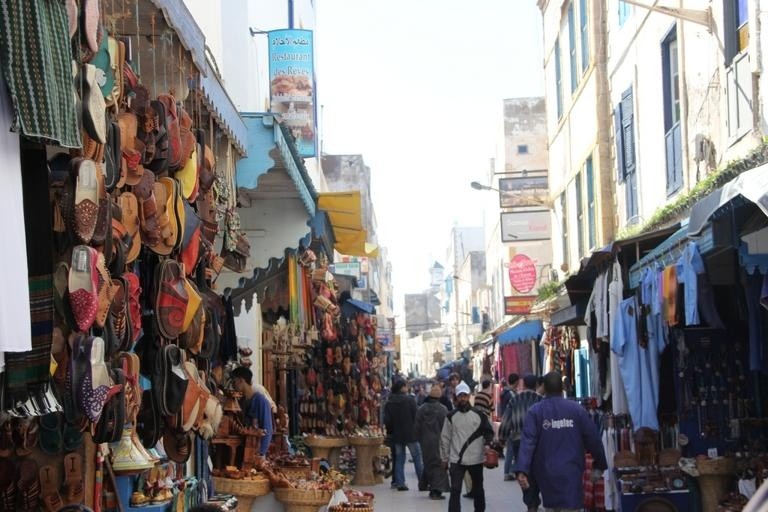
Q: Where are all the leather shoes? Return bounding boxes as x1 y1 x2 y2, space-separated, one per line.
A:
430 494 445 499
398 487 408 491
420 488 430 491
463 493 473 498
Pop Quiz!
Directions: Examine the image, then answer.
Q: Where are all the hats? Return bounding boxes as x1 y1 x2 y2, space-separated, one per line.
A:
454 382 471 397
429 385 442 398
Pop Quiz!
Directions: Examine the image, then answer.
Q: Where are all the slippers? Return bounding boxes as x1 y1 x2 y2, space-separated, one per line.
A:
117 191 142 266
39 464 62 511
180 106 195 158
196 199 218 244
0 461 17 512
164 345 189 416
111 122 121 183
122 272 143 346
15 416 39 456
335 346 342 363
325 348 334 365
177 104 195 165
181 350 203 425
106 36 121 106
62 453 86 507
180 228 200 275
163 413 192 463
181 193 201 248
37 412 65 458
148 182 174 255
158 177 178 250
103 123 116 188
134 168 161 248
115 155 128 189
69 129 97 160
142 131 156 165
197 127 216 201
142 389 156 448
342 357 350 376
129 352 143 420
118 40 138 108
148 100 169 176
60 408 86 451
108 367 124 442
0 419 17 458
91 174 109 244
83 0 100 53
155 260 191 341
119 112 144 185
93 367 116 443
94 144 104 162
89 31 115 96
188 142 201 203
173 177 185 250
64 0 78 39
173 144 197 199
106 192 128 259
130 85 155 144
106 277 126 352
157 95 183 167
119 279 133 351
152 388 165 448
178 264 203 335
193 371 210 431
94 248 122 328
119 352 135 424
16 459 40 512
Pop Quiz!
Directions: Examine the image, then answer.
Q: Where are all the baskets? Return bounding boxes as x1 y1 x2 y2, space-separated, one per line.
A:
329 503 373 512
349 437 384 486
305 438 348 458
213 477 272 511
691 459 735 512
273 487 332 512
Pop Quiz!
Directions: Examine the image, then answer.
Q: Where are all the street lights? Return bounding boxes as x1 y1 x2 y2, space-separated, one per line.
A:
470 181 556 209
453 275 492 290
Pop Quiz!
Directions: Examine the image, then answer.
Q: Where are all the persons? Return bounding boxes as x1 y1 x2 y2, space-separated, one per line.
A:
472 380 494 449
498 374 518 481
383 390 407 488
232 368 274 458
417 385 450 500
517 373 608 511
441 375 458 409
438 378 494 511
499 378 543 511
386 381 423 490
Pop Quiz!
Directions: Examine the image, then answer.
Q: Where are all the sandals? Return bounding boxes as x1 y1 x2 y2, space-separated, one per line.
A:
67 246 100 333
74 63 110 144
62 335 84 424
79 336 111 425
72 157 102 244
